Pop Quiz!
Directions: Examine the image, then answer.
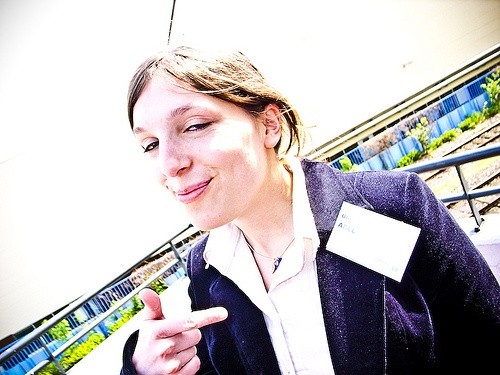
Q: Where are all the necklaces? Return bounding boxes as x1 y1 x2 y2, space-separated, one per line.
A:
241 232 298 276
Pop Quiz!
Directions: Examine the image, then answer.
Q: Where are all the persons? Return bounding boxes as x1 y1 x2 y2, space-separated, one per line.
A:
117 44 500 375
